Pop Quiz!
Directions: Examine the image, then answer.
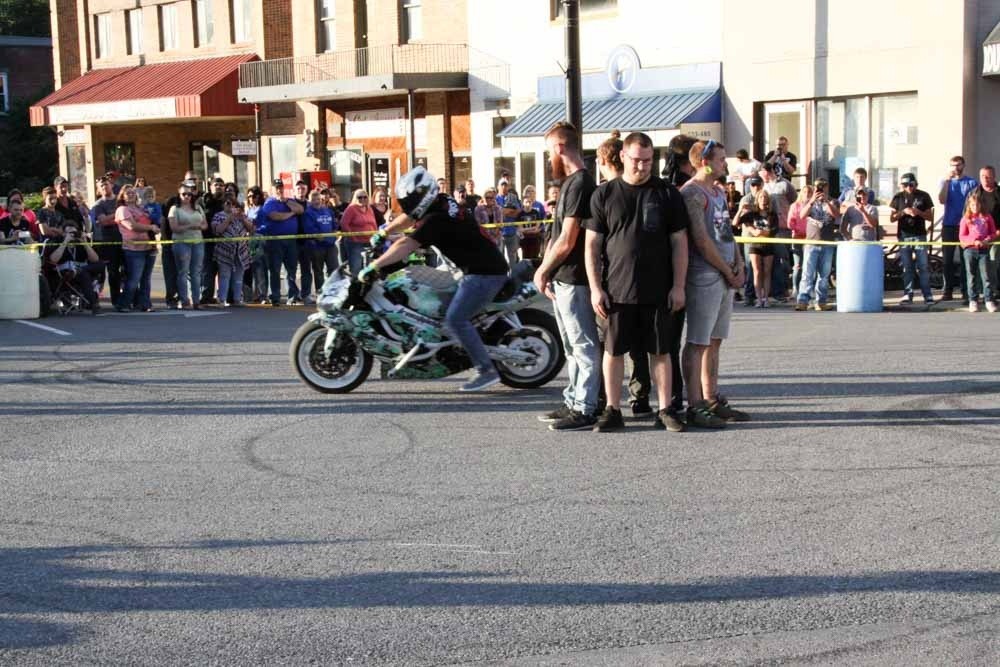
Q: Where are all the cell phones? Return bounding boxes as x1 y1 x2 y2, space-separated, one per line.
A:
856 197 861 204
69 231 81 238
232 208 240 214
818 184 824 192
18 230 31 238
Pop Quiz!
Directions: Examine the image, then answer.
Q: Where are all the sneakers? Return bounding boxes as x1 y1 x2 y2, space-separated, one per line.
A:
537 390 753 434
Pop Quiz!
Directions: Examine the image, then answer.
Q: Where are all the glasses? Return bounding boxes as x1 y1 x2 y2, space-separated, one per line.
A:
357 196 368 200
751 183 762 187
701 138 717 161
247 194 257 199
900 183 914 188
179 192 192 197
226 201 236 206
624 150 654 166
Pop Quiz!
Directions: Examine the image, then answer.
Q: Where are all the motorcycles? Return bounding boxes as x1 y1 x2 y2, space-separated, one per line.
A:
288 230 567 389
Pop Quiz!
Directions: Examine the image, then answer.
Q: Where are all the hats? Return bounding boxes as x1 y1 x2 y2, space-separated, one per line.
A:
454 184 465 192
751 177 763 184
855 186 870 195
498 178 509 186
54 176 69 185
272 180 283 187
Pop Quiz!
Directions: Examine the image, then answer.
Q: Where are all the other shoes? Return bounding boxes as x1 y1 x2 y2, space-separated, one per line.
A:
459 368 500 392
736 285 1000 313
40 289 322 315
150 249 159 256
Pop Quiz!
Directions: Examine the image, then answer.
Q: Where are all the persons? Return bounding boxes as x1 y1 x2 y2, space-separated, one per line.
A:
728 137 877 311
454 170 562 269
0 170 446 317
533 122 751 431
938 156 1000 312
889 173 935 305
359 166 510 391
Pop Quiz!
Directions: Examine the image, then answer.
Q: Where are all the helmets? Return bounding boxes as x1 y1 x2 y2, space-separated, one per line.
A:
394 166 440 221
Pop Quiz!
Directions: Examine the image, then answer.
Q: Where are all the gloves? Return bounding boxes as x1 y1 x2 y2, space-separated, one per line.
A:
369 229 389 255
358 261 380 284
